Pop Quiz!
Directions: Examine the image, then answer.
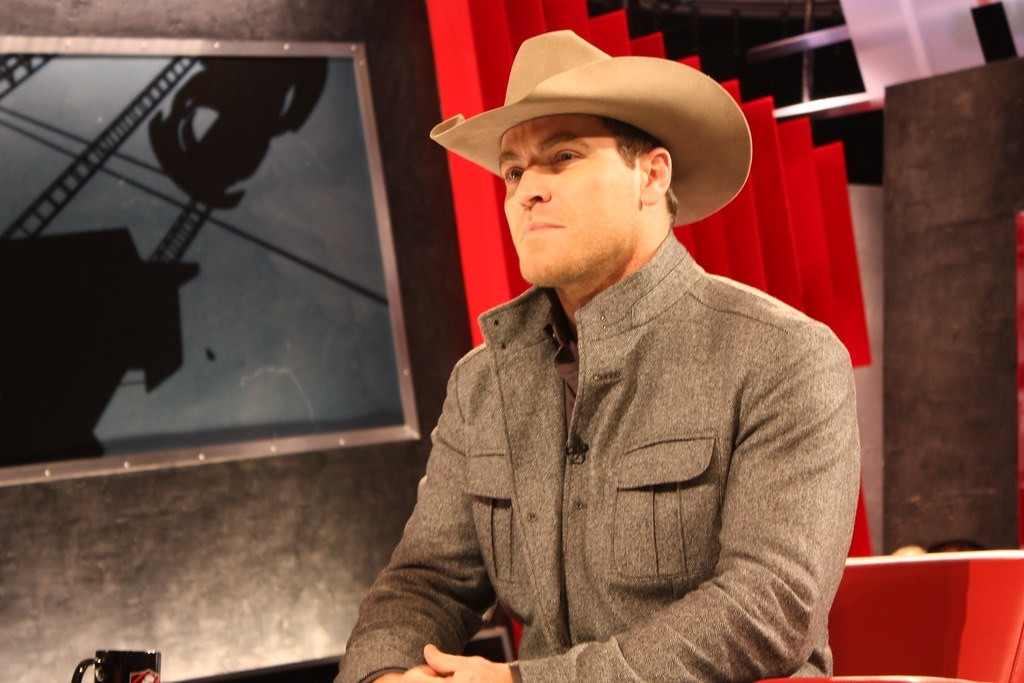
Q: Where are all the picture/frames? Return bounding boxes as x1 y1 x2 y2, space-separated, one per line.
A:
0 34 420 486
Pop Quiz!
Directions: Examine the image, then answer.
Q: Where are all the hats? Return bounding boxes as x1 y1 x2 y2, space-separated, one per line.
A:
430 30 752 226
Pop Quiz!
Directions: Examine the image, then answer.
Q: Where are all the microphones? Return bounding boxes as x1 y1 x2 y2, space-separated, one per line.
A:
570 435 588 455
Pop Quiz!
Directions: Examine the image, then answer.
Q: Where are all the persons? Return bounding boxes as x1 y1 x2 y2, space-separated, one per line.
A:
336 29 862 683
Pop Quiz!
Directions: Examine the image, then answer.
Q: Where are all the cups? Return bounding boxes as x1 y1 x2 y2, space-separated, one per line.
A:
70 648 162 683
